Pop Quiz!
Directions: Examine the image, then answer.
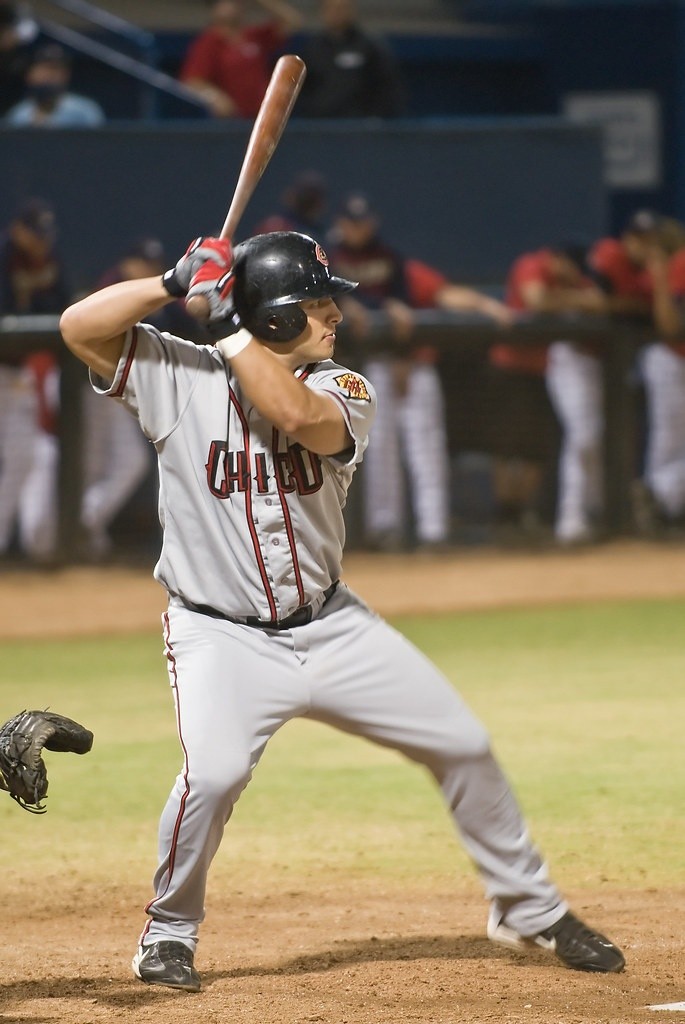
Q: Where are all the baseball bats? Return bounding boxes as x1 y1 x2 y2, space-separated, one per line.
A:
185 51 308 321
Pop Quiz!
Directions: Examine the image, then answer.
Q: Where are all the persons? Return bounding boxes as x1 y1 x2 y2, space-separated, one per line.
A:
487 210 684 545
180 0 391 119
0 0 103 128
58 231 628 993
0 179 517 568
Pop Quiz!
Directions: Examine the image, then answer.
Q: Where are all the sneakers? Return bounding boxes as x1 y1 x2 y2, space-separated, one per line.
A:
487 897 626 973
132 940 201 992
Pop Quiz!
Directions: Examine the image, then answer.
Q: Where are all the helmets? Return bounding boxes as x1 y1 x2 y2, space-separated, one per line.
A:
231 230 360 344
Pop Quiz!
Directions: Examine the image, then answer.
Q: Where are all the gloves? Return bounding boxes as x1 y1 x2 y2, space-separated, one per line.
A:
184 258 244 341
163 236 234 298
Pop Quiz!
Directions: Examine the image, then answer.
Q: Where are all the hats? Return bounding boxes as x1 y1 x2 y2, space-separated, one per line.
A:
619 207 664 238
120 233 171 273
333 189 379 221
552 238 593 275
12 194 58 237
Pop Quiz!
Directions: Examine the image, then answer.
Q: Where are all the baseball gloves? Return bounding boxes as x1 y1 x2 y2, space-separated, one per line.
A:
1 704 96 817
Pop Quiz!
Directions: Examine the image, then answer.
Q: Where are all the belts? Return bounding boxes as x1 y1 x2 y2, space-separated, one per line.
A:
168 580 341 631
